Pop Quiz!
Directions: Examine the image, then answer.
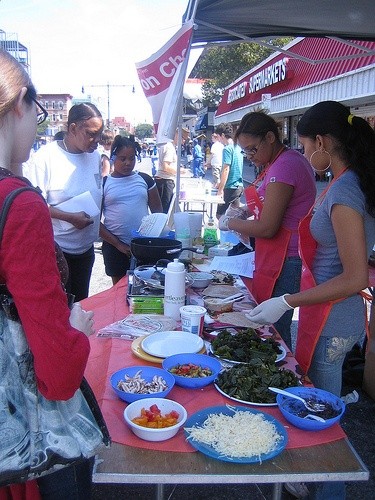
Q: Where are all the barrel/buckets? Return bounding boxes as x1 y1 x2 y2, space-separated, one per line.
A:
172 211 203 245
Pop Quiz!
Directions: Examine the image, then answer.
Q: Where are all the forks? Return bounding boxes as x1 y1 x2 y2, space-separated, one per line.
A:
269 387 325 412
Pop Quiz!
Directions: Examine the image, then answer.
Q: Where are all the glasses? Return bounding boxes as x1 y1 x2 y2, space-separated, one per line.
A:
27 91 48 125
239 138 263 158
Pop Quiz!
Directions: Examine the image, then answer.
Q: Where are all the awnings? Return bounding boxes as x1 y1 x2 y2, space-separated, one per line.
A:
182 0 375 44
194 114 209 133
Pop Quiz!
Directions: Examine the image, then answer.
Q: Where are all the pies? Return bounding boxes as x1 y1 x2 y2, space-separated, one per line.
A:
216 311 265 329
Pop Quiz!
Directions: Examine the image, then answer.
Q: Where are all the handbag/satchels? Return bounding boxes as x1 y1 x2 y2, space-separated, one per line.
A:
0 186 111 489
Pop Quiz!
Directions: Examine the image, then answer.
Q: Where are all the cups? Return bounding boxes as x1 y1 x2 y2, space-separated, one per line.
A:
175 234 193 260
180 305 207 339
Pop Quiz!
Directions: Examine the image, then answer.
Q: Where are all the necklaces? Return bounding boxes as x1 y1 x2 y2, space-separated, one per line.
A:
62 138 69 152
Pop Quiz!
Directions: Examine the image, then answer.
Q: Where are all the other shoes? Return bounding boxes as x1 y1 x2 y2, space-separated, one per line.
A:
191 175 197 178
202 174 205 178
284 482 310 500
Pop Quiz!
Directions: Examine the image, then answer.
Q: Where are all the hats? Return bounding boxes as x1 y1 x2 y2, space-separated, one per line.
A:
193 139 198 143
176 127 191 140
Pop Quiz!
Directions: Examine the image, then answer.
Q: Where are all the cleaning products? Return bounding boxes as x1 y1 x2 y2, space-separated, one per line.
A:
203 217 218 244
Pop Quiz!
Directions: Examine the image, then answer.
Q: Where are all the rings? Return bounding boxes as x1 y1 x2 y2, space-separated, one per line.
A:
90 319 96 325
73 303 82 309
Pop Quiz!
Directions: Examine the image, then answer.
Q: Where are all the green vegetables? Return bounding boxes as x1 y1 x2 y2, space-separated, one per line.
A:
211 328 306 403
209 270 233 283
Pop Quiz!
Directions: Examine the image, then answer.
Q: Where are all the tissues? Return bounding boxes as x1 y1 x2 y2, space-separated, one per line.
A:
208 241 237 258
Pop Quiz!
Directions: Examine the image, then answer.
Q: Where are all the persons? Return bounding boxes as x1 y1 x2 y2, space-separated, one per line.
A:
218 111 317 354
245 100 375 398
24 102 114 304
99 135 165 287
19 123 244 255
0 50 95 500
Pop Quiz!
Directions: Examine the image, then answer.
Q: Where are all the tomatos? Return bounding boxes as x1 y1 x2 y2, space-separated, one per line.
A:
177 363 196 375
133 404 179 428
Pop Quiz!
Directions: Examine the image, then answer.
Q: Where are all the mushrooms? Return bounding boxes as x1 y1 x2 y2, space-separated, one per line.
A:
117 370 169 394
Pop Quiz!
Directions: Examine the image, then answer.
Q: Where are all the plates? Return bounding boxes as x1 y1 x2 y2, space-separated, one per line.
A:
141 331 204 358
214 365 303 405
210 338 286 364
185 405 287 463
131 334 206 363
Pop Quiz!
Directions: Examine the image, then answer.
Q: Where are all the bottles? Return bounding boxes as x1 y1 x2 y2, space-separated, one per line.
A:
204 217 218 255
164 259 186 322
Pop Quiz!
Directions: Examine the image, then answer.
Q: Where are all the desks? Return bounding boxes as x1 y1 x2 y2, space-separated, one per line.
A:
92 248 370 500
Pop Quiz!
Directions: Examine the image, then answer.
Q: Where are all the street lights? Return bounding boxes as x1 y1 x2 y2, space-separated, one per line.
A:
81 81 136 132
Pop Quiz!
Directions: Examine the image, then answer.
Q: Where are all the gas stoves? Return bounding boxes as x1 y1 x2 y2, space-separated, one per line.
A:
126 252 190 271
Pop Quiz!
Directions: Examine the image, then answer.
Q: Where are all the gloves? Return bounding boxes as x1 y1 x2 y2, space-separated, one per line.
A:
244 293 295 328
218 215 234 231
237 205 253 218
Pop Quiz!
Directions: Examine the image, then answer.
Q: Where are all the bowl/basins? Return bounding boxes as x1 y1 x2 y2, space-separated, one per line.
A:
220 229 242 244
123 397 188 442
204 294 233 315
188 273 214 288
162 353 222 389
110 366 176 403
276 387 345 430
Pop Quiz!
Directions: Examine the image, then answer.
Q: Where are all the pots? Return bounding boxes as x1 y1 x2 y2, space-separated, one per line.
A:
130 236 205 263
